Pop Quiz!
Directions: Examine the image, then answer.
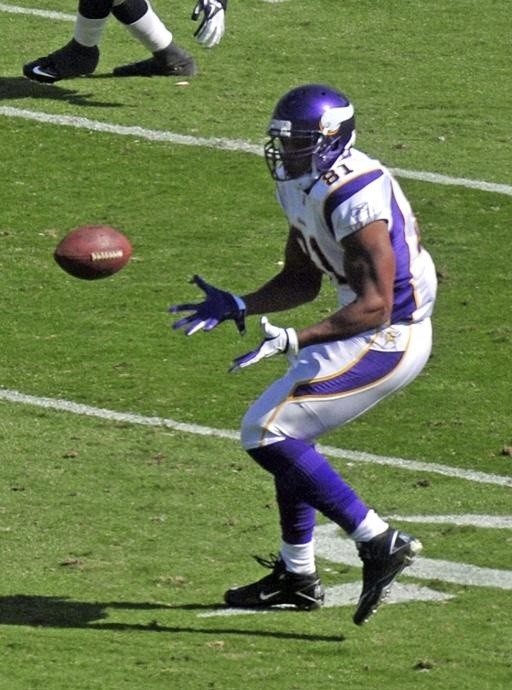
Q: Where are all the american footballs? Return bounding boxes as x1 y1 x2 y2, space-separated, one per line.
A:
53 224 132 278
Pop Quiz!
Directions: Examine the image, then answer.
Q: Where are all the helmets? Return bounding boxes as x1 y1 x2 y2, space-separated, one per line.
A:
264 84 356 181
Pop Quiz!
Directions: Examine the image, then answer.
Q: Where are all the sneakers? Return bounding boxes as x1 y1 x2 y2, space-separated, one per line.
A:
23 39 99 83
354 526 423 625
224 551 324 610
113 49 195 75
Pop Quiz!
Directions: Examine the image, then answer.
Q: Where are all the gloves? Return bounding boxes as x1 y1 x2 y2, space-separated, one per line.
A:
169 275 246 336
192 0 224 47
228 316 298 374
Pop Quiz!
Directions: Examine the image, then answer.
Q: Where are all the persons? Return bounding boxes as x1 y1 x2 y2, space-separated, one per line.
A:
168 84 439 626
21 0 228 83
